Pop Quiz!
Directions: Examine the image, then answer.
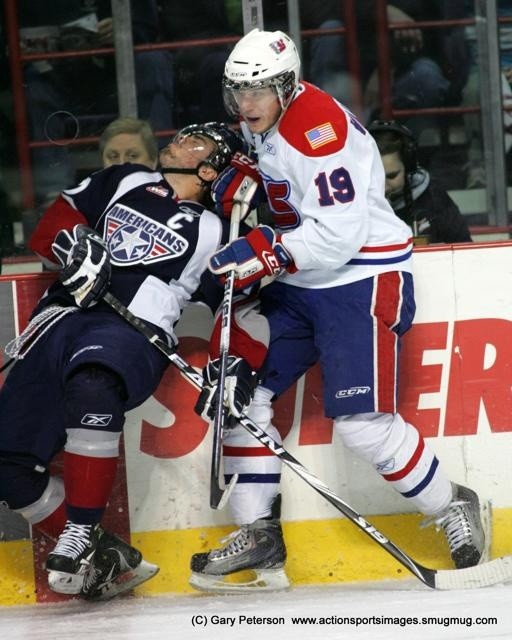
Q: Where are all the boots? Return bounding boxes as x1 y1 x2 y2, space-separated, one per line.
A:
46 520 100 573
80 525 143 599
189 518 287 575
432 481 485 568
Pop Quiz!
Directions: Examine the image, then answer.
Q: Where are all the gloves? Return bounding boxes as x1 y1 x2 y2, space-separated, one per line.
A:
207 224 291 290
55 224 111 310
193 355 257 429
210 150 262 221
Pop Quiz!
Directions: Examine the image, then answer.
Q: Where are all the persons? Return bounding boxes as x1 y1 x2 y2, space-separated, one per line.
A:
190 29 486 574
1 1 512 244
0 122 270 602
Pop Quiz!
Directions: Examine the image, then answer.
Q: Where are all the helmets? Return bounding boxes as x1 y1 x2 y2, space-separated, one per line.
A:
221 28 301 110
178 120 243 172
